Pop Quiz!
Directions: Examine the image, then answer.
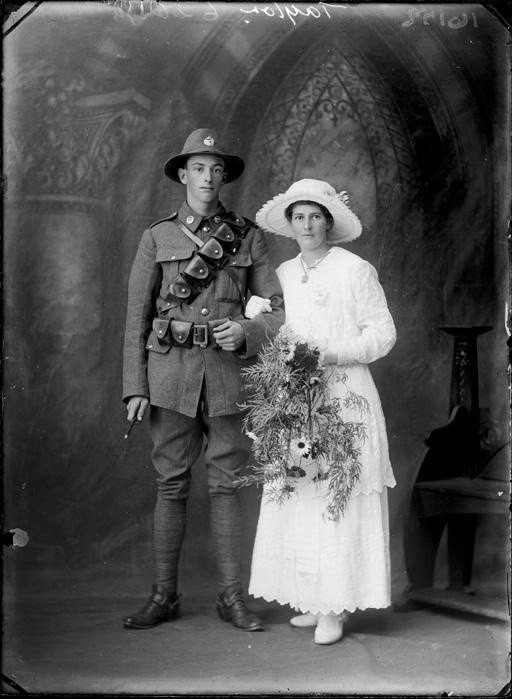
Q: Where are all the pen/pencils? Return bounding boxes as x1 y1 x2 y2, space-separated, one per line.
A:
123 417 138 440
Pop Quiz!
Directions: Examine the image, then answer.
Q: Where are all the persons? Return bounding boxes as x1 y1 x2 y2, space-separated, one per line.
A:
243 180 397 646
120 127 285 632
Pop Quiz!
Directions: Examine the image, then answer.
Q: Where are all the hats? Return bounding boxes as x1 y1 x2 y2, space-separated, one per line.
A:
256 178 362 245
163 129 245 184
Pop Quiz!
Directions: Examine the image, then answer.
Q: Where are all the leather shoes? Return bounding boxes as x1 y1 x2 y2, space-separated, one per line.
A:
290 611 316 626
314 614 343 645
123 583 181 629
216 586 263 631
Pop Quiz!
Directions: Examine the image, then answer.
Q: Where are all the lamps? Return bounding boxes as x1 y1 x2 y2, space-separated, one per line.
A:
433 276 493 593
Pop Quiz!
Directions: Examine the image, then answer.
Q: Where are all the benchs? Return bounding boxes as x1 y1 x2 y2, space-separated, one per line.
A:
391 405 512 622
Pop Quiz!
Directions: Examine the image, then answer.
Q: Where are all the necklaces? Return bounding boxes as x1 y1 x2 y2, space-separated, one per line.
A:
299 254 327 284
